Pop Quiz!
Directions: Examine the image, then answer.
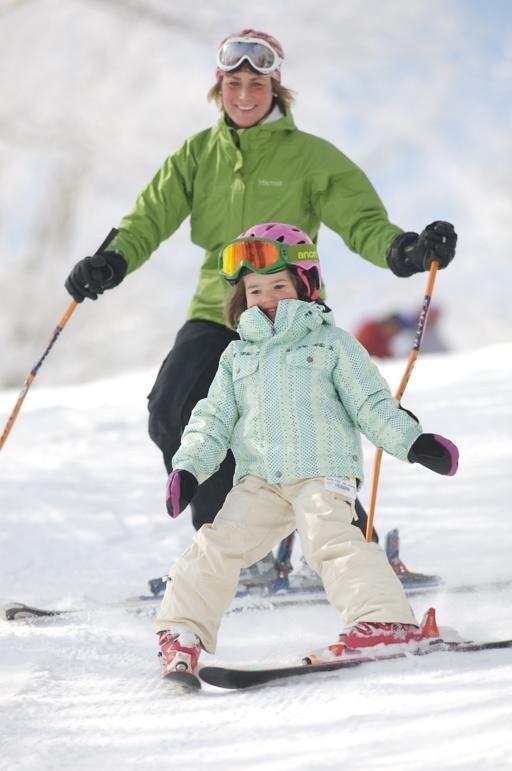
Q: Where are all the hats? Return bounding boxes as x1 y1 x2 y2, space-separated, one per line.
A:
214 28 284 78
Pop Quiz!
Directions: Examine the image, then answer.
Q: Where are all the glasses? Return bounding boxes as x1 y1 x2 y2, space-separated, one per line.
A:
216 37 280 75
216 238 287 281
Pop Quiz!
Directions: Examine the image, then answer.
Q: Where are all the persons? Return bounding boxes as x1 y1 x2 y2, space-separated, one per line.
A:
61 27 459 595
352 311 406 361
415 305 449 355
154 219 461 681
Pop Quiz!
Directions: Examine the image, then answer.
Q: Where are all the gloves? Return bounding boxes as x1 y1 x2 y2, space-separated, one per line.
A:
409 433 459 476
384 220 457 278
164 470 198 518
64 251 128 304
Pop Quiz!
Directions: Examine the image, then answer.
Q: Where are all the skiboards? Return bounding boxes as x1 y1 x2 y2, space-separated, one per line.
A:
4 576 512 621
159 638 510 692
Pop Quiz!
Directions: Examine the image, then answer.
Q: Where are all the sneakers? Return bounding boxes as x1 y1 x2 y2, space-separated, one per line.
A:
156 630 202 673
239 552 278 586
339 620 420 649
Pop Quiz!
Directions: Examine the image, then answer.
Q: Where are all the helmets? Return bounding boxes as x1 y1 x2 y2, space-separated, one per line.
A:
235 222 322 289
